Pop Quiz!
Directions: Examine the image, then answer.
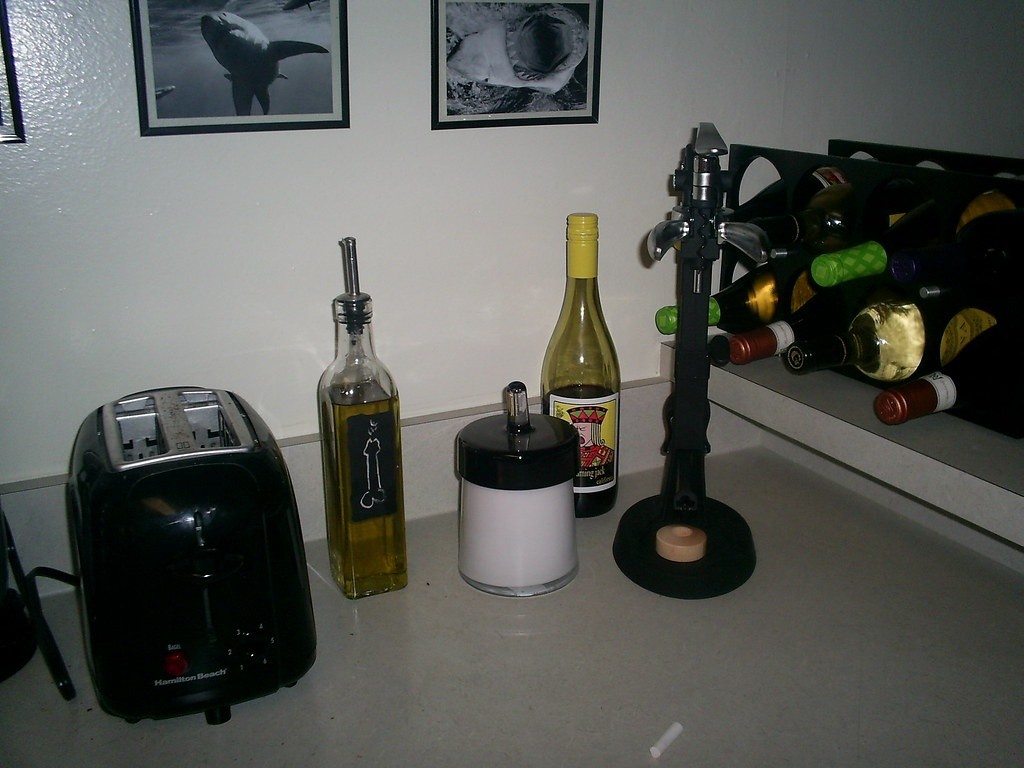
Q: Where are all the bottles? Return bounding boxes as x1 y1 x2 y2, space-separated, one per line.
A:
456 385 583 597
319 237 409 598
541 212 620 516
658 159 1024 437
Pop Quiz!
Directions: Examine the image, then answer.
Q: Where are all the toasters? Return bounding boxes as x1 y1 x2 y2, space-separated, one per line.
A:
64 386 317 726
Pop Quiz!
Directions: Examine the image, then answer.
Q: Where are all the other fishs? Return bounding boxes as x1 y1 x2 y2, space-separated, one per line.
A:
199 10 330 116
448 7 588 93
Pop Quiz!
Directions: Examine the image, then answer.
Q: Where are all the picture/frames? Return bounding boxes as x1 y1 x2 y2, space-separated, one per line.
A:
430 0 604 130
129 0 350 136
0 1 26 144
